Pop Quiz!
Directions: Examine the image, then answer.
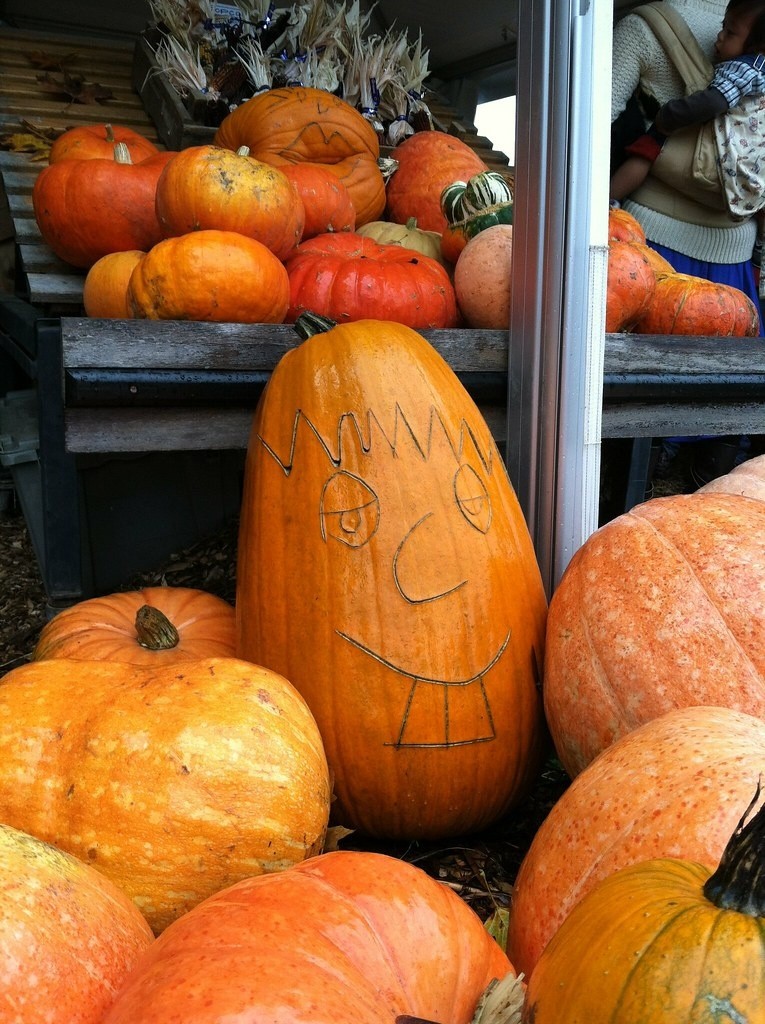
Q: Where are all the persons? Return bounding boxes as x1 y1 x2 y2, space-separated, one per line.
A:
608 0 765 480
609 1 765 224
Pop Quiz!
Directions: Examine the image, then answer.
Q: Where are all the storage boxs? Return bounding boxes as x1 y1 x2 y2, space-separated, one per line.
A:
132 32 466 158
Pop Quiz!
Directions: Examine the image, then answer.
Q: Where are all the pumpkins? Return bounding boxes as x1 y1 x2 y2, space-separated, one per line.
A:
31 79 759 336
0 319 765 1024
